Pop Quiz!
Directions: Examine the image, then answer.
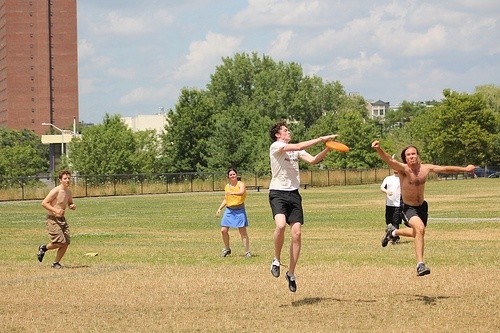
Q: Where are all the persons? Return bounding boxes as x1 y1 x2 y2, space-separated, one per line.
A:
216 168 254 259
380 155 402 244
267 122 339 292
371 140 476 277
36 171 76 270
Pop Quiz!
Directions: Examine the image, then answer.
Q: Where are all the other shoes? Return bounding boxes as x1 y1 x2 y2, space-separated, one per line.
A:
390 236 400 244
246 251 251 258
221 249 231 257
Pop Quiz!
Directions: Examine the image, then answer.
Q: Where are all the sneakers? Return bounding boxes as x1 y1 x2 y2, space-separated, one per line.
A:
51 262 63 269
271 257 280 277
417 263 430 276
38 244 48 262
382 223 395 247
285 271 296 292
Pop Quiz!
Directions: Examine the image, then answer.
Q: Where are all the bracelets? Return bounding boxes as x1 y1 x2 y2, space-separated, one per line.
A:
320 137 325 143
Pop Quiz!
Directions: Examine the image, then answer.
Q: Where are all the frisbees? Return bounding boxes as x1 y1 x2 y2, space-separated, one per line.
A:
325 141 350 153
